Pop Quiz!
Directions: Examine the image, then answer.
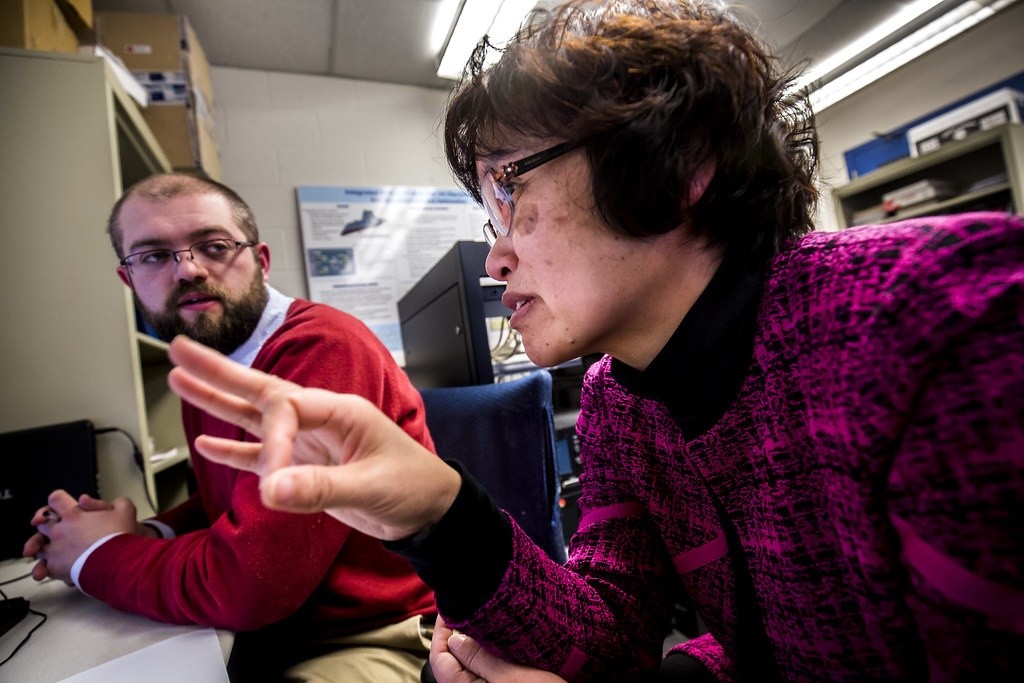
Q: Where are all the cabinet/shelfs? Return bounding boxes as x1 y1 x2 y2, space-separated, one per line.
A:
0 48 191 521
830 123 1024 228
397 240 583 505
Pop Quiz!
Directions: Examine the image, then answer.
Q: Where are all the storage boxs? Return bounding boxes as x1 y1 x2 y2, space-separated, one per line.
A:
0 0 79 51
97 10 213 102
138 84 218 180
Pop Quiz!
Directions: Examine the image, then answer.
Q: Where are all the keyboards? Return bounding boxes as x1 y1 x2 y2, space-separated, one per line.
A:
0 597 31 637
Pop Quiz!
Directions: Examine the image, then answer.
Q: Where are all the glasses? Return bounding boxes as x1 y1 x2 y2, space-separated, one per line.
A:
481 137 585 251
121 237 254 278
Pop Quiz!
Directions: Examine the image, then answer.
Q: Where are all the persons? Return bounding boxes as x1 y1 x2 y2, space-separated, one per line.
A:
22 172 440 683
165 0 1024 683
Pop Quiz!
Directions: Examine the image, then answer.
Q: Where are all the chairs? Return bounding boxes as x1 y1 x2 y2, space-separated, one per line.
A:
416 367 566 567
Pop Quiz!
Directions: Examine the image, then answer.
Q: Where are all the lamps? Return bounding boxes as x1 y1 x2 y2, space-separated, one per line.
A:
775 0 1019 128
433 0 542 81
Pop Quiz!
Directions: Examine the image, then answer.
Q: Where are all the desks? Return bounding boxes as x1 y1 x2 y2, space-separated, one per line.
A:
0 554 230 683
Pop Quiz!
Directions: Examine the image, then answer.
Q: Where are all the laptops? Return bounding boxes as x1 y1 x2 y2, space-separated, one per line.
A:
0 419 102 563
